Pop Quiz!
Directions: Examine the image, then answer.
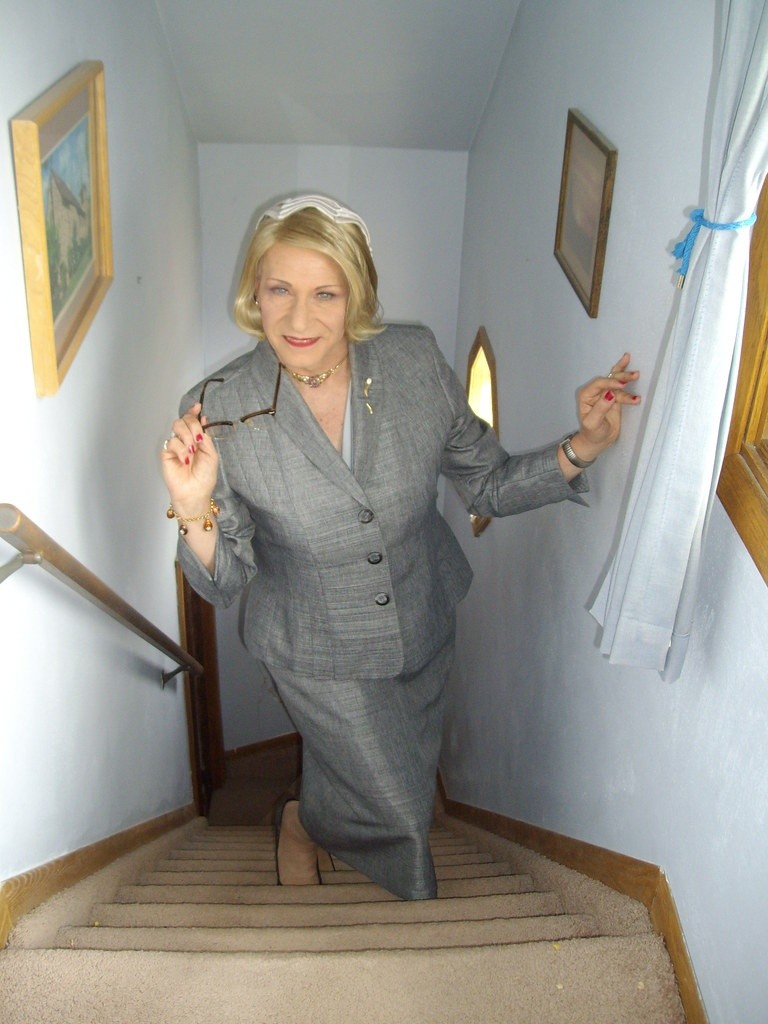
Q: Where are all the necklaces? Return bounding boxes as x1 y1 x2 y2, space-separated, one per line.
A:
281 349 355 390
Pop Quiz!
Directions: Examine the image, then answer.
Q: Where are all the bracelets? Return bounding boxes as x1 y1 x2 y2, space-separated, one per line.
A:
166 496 223 536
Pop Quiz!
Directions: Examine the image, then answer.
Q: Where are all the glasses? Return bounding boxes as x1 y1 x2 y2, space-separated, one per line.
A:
196 364 282 438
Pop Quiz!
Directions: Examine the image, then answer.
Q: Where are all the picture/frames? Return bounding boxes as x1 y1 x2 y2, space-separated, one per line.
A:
8 60 114 395
552 107 618 320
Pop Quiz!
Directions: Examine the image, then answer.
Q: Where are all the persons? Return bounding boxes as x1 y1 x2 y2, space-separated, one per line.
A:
157 195 645 901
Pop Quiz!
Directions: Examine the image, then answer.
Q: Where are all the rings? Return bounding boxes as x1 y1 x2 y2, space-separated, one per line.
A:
163 438 170 452
170 430 182 441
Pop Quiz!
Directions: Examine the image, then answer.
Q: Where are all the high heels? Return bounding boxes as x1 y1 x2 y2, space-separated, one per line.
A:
272 797 325 887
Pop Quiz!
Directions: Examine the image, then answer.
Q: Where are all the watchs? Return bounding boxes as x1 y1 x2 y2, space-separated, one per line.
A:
557 429 599 469
